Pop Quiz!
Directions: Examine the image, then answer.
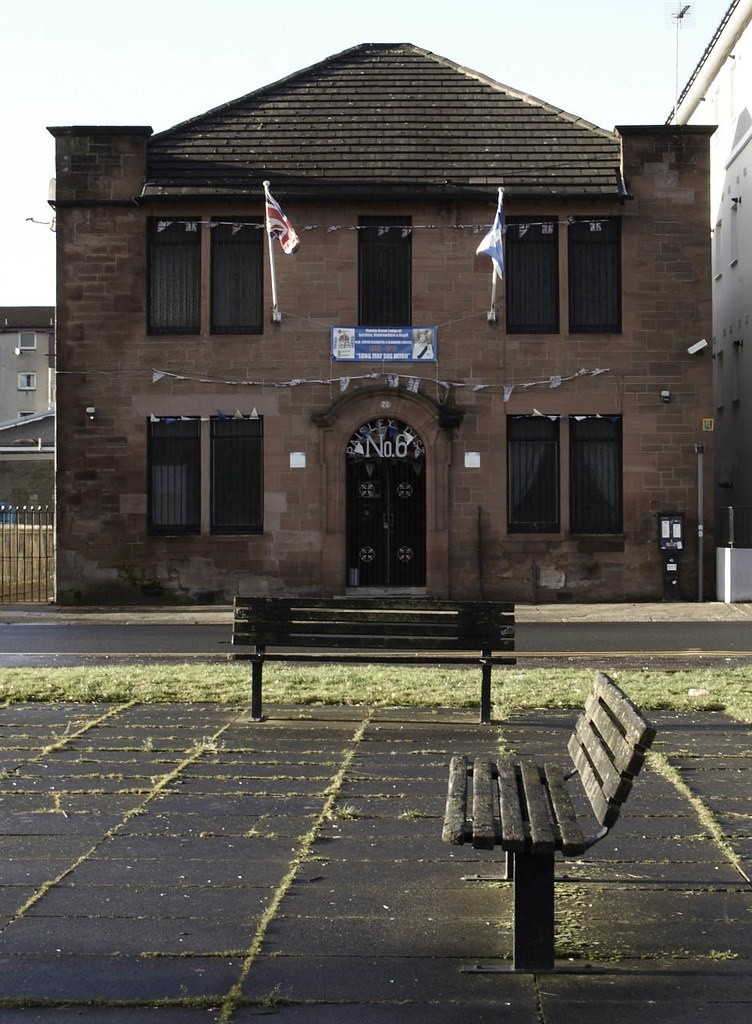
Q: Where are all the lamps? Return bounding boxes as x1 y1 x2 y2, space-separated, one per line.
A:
87 408 96 421
661 391 670 403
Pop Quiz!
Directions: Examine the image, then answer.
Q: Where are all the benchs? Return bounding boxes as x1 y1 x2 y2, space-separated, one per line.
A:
441 673 656 975
227 596 516 727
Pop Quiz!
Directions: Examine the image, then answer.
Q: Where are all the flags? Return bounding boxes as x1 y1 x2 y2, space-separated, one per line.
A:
263 179 300 255
474 185 507 280
